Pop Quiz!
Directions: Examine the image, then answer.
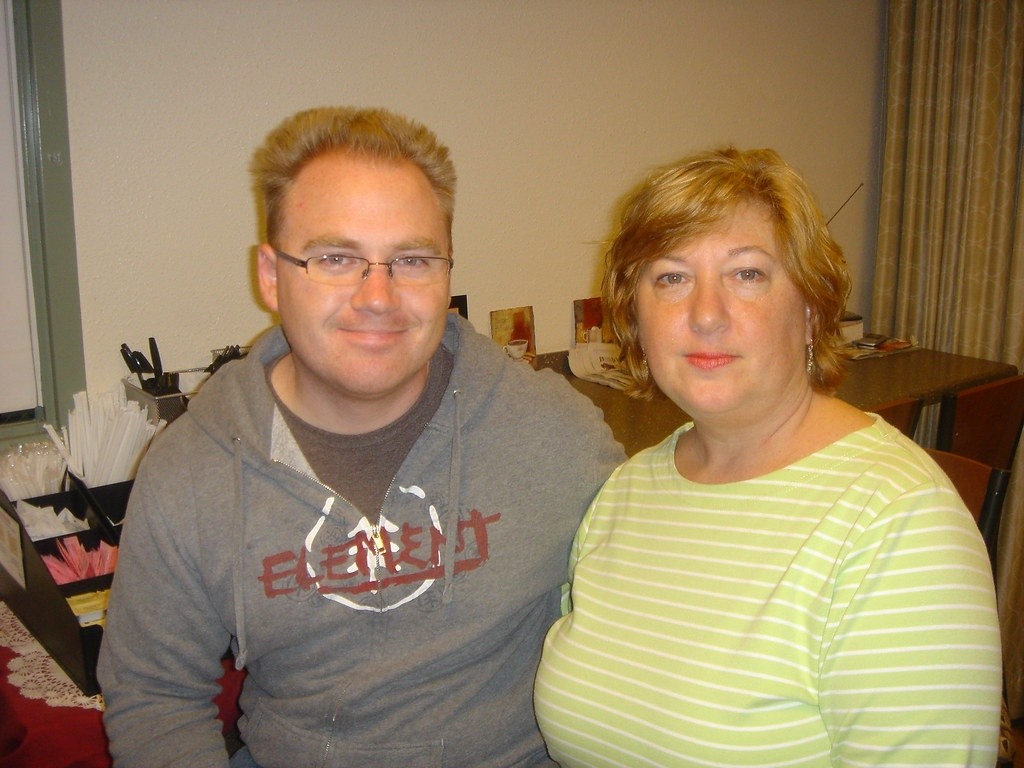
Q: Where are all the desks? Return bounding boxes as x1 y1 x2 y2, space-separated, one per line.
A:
560 350 1018 459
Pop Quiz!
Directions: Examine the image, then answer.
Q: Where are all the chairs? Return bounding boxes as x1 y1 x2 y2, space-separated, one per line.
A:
919 446 1008 546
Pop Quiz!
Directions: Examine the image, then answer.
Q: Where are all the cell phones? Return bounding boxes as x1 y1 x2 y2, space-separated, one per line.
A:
856 333 887 350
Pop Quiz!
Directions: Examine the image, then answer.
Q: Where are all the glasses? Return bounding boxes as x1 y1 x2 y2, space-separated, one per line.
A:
268 247 454 286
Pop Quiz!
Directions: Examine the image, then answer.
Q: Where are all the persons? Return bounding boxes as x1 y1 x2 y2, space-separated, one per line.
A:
96 106 632 768
533 146 1004 768
